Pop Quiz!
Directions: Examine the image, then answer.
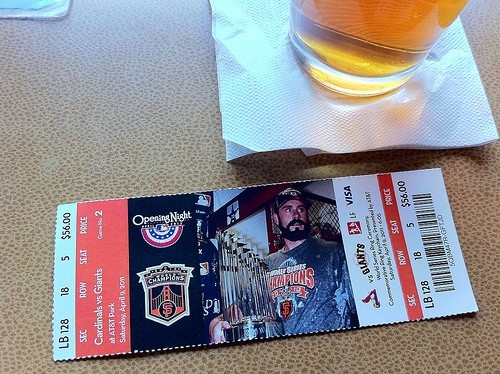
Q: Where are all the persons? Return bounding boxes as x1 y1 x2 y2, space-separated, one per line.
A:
206 188 359 345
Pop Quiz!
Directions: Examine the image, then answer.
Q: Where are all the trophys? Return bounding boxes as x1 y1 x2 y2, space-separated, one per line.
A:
214 226 285 343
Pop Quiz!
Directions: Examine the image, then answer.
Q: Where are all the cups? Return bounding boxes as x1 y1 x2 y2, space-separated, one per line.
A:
288 0 474 100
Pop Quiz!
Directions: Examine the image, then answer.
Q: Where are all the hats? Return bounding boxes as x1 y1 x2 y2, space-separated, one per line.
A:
276 186 312 210
